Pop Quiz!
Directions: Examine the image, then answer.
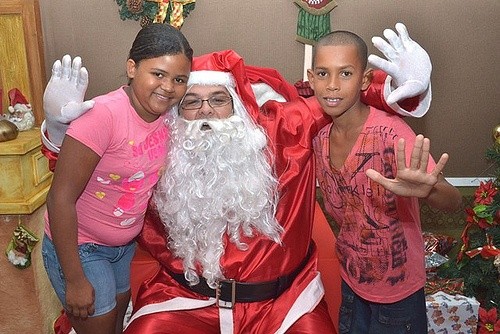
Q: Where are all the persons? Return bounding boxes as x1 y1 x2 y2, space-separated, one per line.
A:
41 23 193 334
45 23 433 334
307 31 462 334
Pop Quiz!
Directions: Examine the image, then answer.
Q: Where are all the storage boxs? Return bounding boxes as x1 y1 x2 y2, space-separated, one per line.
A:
423 290 480 334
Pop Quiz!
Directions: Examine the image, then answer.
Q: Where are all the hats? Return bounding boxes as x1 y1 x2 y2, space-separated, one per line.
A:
187 49 259 121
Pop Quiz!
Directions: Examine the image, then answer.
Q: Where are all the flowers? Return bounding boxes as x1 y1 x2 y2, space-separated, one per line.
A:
456 179 500 231
477 306 500 334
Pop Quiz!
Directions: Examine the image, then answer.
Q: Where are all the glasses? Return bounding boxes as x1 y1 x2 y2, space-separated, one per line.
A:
179 96 232 110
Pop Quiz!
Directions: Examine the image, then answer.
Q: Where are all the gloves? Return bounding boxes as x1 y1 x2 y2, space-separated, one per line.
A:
43 54 95 148
367 22 432 105
